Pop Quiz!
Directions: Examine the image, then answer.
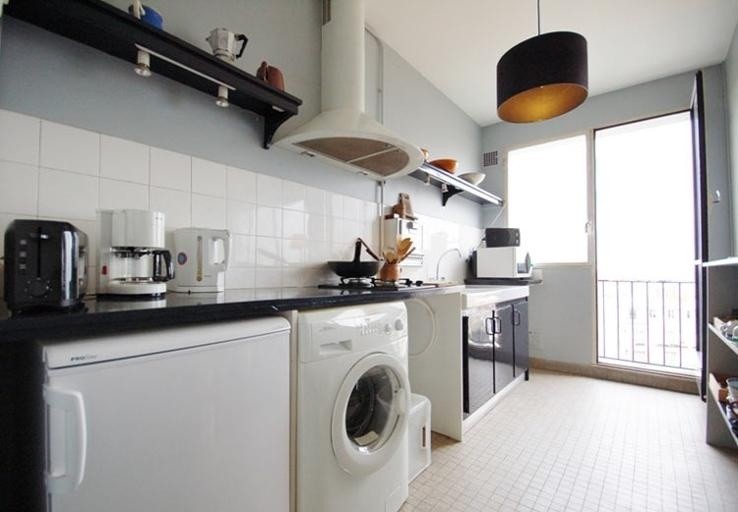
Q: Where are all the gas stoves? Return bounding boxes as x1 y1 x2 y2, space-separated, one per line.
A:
319 278 437 291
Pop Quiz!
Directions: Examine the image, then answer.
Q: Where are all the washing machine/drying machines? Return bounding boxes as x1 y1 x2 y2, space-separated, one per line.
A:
278 300 410 512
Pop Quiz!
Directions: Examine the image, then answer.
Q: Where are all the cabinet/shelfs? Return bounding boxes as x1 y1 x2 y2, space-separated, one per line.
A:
403 300 512 441
705 259 738 449
512 296 529 382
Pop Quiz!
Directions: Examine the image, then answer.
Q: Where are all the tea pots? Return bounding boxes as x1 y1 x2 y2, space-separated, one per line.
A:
205 28 248 64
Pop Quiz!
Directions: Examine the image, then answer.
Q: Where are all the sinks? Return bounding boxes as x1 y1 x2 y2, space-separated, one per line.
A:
446 285 530 312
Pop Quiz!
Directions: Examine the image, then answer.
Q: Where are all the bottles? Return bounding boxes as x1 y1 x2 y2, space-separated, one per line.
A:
718 381 729 402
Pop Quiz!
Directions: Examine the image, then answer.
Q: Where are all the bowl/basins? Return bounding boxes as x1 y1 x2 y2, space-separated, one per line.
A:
457 173 486 185
726 377 738 397
420 149 429 162
429 159 457 174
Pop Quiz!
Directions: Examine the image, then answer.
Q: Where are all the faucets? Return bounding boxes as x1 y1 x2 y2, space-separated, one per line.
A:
436 247 463 281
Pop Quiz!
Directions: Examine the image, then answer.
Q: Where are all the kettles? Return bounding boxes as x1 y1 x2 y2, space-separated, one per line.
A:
172 227 234 295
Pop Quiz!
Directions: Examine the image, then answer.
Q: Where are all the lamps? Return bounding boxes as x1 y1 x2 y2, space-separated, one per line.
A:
496 0 589 124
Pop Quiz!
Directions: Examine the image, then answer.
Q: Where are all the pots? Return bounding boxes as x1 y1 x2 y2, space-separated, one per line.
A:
329 239 385 280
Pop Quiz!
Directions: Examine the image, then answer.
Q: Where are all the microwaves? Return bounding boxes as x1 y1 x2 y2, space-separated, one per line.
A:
475 246 535 277
484 228 520 246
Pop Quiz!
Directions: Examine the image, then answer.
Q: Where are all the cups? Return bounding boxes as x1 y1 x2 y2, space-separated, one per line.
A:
129 2 163 30
720 320 738 339
257 61 283 89
382 261 400 282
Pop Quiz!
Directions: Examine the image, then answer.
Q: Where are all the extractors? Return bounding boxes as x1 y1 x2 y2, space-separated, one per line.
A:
277 2 425 185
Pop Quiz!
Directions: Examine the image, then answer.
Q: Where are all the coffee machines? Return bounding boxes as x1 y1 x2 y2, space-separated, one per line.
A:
97 208 172 300
2 219 91 311
